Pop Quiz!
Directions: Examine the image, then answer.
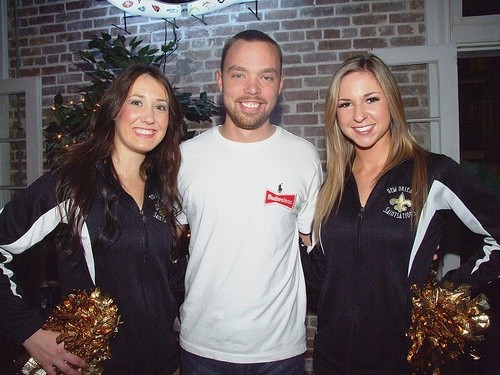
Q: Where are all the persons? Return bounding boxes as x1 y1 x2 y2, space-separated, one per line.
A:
0 63 190 375
299 55 500 375
175 30 324 374
